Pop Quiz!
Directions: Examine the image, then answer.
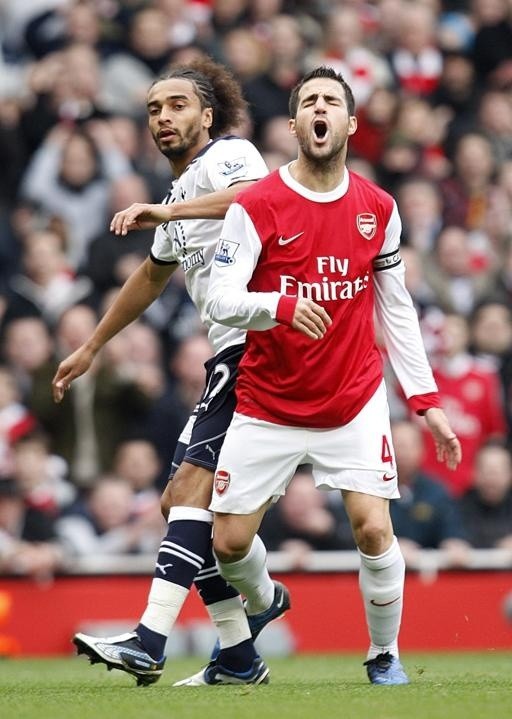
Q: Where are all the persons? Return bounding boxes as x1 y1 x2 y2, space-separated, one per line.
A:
1 0 511 581
49 59 271 686
205 68 462 686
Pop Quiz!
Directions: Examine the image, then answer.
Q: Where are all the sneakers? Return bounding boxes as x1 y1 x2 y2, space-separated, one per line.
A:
362 652 410 685
173 581 291 687
72 631 166 683
